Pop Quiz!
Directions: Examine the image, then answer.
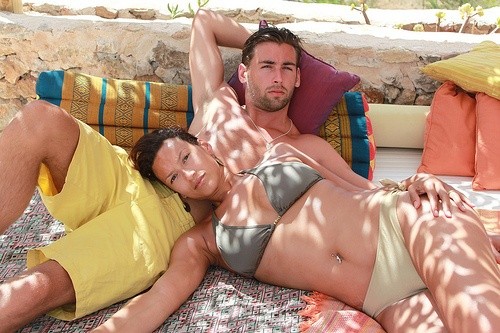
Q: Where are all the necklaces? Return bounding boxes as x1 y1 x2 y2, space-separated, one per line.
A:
243 104 292 151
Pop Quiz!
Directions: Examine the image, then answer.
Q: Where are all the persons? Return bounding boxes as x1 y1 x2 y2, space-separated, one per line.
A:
86 125 500 333
0 8 380 333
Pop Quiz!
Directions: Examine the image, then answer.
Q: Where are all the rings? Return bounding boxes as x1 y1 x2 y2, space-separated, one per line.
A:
447 190 455 194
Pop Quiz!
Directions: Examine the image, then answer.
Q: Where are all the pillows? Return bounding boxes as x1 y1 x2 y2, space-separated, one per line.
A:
471 92 500 190
417 81 475 178
36 71 375 181
422 41 500 100
366 103 430 149
227 19 360 136
